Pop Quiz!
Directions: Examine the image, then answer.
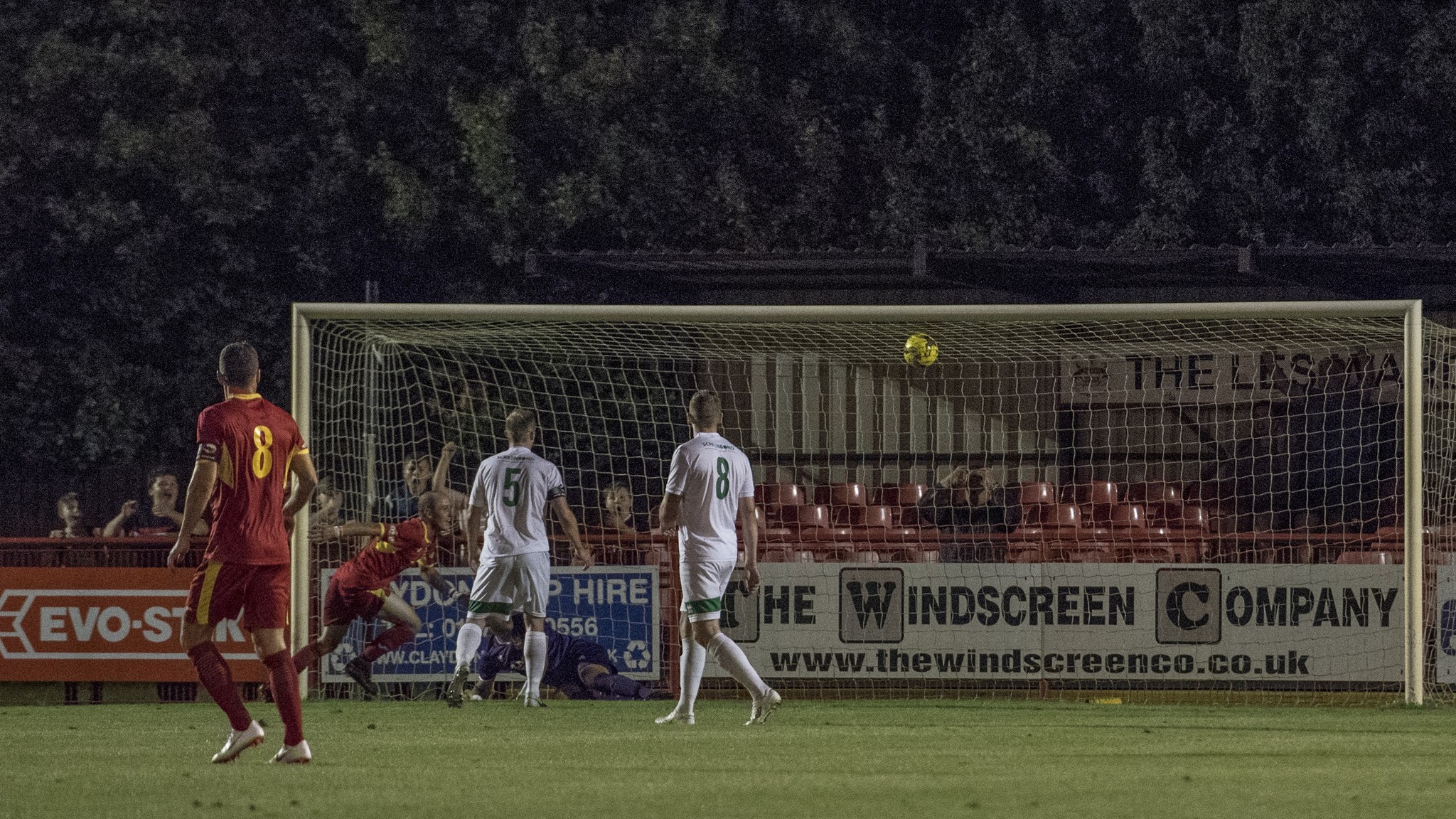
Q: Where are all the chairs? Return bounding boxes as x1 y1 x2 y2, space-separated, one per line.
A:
649 483 1456 564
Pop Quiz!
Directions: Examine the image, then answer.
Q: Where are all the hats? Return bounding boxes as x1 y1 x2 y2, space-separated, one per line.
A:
317 475 341 492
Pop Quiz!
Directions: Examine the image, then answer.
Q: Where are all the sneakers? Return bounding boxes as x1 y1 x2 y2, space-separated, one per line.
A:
654 711 695 726
268 740 312 764
342 653 379 696
211 720 265 764
524 691 549 709
742 688 781 726
257 680 276 704
445 661 471 709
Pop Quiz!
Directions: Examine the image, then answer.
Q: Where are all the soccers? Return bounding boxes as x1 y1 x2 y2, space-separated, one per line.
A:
904 334 939 369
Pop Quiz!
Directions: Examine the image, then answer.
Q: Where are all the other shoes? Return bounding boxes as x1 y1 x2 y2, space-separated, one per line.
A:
643 691 674 700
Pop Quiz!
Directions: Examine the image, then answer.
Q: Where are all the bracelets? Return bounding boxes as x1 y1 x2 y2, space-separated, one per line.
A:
334 526 343 538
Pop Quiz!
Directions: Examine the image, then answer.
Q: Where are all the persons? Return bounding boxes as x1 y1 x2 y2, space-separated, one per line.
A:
167 344 313 764
917 466 1023 563
654 391 782 726
258 490 471 705
436 408 593 706
52 474 650 707
465 611 675 700
